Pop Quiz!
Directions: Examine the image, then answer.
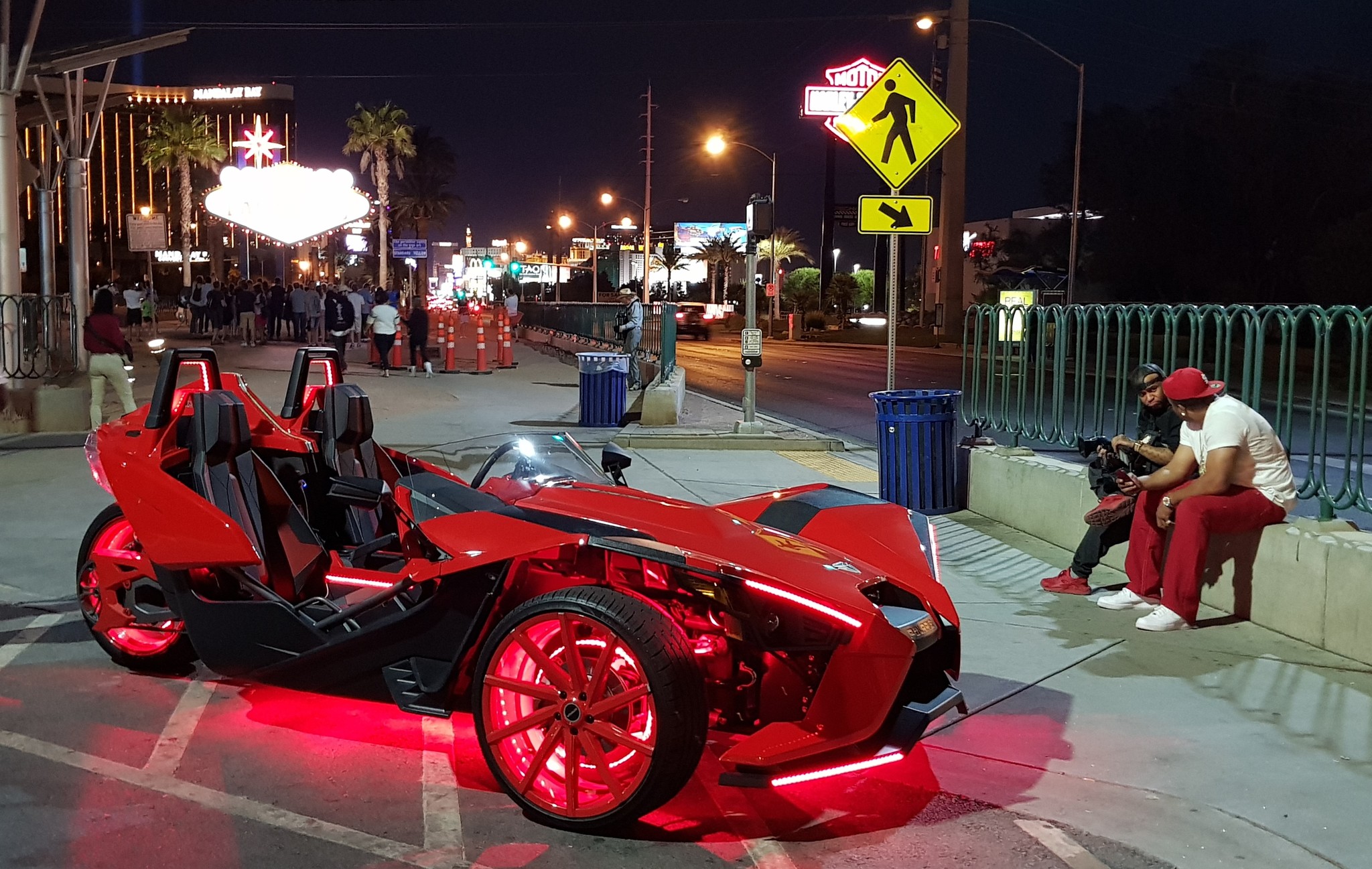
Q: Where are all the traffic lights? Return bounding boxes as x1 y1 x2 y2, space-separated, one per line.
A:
485 255 491 268
512 256 520 272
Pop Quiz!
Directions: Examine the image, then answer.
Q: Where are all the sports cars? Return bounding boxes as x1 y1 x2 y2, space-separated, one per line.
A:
74 345 968 831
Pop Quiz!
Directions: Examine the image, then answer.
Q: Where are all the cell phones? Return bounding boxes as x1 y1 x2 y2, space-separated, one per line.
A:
1115 470 1141 494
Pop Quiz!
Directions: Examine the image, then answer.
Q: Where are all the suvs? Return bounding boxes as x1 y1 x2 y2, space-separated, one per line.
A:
673 301 707 334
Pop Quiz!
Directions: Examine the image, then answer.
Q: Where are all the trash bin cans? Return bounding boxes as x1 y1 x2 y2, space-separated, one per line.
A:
870 388 964 517
574 351 630 428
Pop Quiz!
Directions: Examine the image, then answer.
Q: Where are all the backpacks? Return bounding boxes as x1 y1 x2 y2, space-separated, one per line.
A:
614 310 628 341
192 283 202 302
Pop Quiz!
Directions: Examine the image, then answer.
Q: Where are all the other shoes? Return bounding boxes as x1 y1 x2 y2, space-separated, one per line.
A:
128 337 145 342
188 330 362 350
378 369 390 377
628 385 638 391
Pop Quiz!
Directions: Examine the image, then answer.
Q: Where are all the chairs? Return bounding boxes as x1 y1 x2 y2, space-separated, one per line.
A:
318 381 397 548
187 388 300 606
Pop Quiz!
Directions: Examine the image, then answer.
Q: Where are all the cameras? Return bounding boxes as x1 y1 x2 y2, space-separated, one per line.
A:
615 312 626 318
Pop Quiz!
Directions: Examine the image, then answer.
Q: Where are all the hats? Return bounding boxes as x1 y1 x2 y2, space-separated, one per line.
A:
1128 363 1167 396
616 288 637 300
1162 367 1226 400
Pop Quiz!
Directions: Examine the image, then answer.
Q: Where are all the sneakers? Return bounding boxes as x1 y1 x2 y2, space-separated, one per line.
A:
1040 567 1092 596
1135 603 1191 631
1083 494 1136 527
1097 586 1161 611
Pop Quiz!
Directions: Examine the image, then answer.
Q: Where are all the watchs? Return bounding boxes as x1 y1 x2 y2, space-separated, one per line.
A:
1162 494 1175 510
1133 441 1144 452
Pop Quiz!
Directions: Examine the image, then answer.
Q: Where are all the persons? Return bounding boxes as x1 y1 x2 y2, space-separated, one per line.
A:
122 271 521 385
613 287 644 391
1096 369 1297 632
83 289 138 432
1039 363 1200 596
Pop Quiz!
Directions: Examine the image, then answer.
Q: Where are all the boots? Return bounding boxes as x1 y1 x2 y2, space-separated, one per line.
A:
408 365 417 377
423 361 436 377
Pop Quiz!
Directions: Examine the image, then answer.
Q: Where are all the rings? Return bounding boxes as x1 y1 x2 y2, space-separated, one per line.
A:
1166 520 1171 523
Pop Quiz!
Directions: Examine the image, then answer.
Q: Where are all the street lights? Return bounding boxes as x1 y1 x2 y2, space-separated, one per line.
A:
914 12 1085 307
600 192 693 303
706 135 777 340
559 215 631 304
